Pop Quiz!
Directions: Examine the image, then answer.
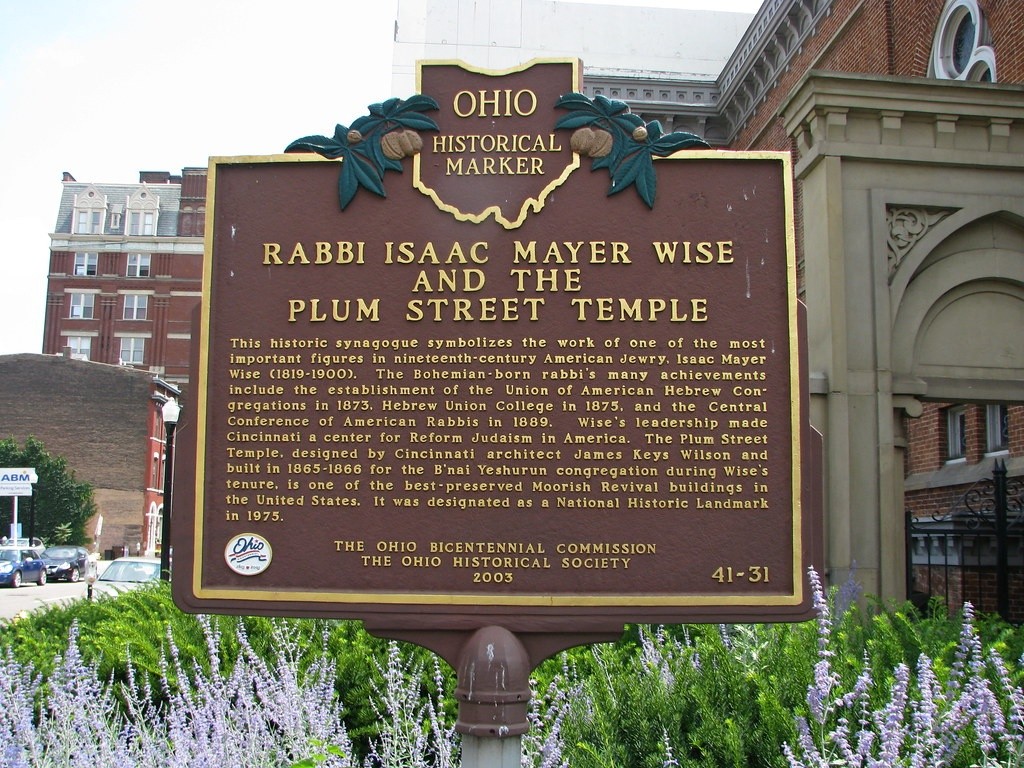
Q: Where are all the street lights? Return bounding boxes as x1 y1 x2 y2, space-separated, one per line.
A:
160 396 181 583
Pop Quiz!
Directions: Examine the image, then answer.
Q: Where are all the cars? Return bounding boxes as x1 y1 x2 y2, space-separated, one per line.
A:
5 537 46 557
81 557 172 603
40 546 89 582
0 545 47 587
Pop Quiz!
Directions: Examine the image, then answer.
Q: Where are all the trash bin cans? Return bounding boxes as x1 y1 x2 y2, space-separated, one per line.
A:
112 546 124 560
105 550 113 560
